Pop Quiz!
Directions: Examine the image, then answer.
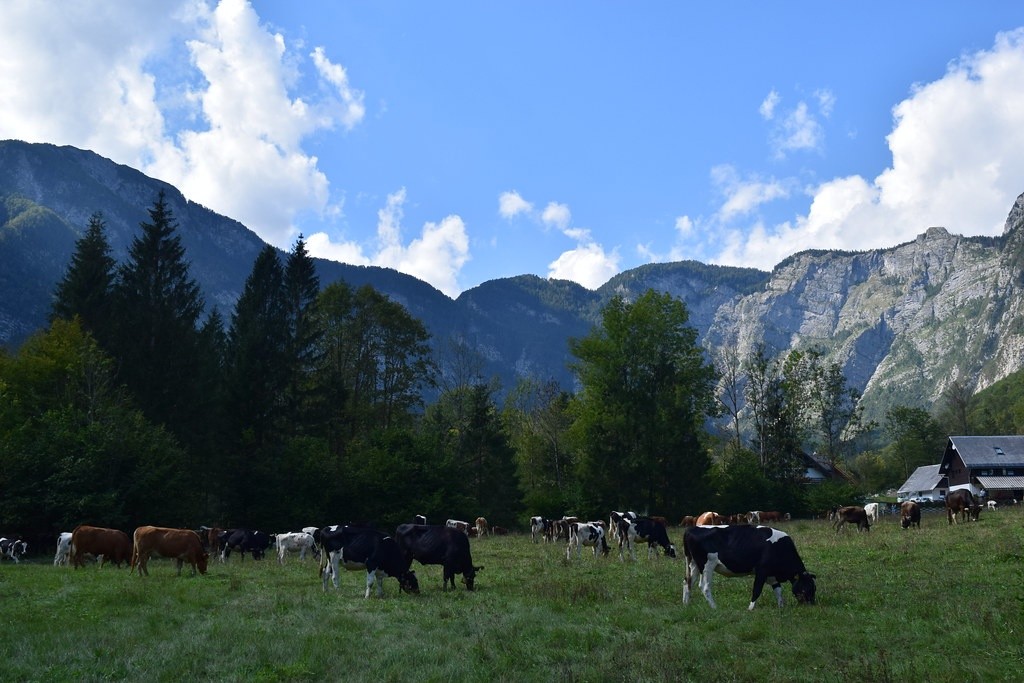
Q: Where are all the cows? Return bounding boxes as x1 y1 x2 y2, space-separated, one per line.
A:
944 488 982 524
986 500 998 510
0 537 29 564
53 526 209 579
529 510 677 562
826 502 880 533
193 526 321 567
901 501 921 531
679 511 816 610
319 515 513 600
